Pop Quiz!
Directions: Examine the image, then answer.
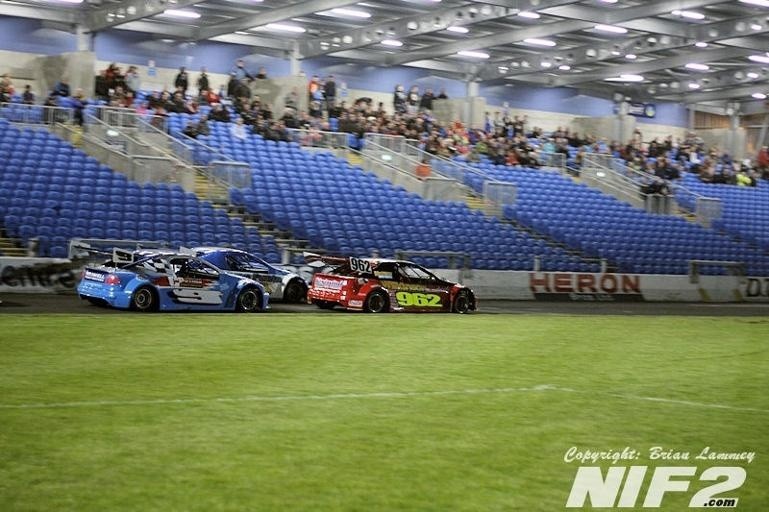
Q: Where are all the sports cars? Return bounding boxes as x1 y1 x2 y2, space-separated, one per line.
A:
73 244 272 313
133 243 310 304
303 251 476 314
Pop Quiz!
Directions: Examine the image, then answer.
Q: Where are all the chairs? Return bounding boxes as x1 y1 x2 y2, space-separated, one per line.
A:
1 73 769 283
